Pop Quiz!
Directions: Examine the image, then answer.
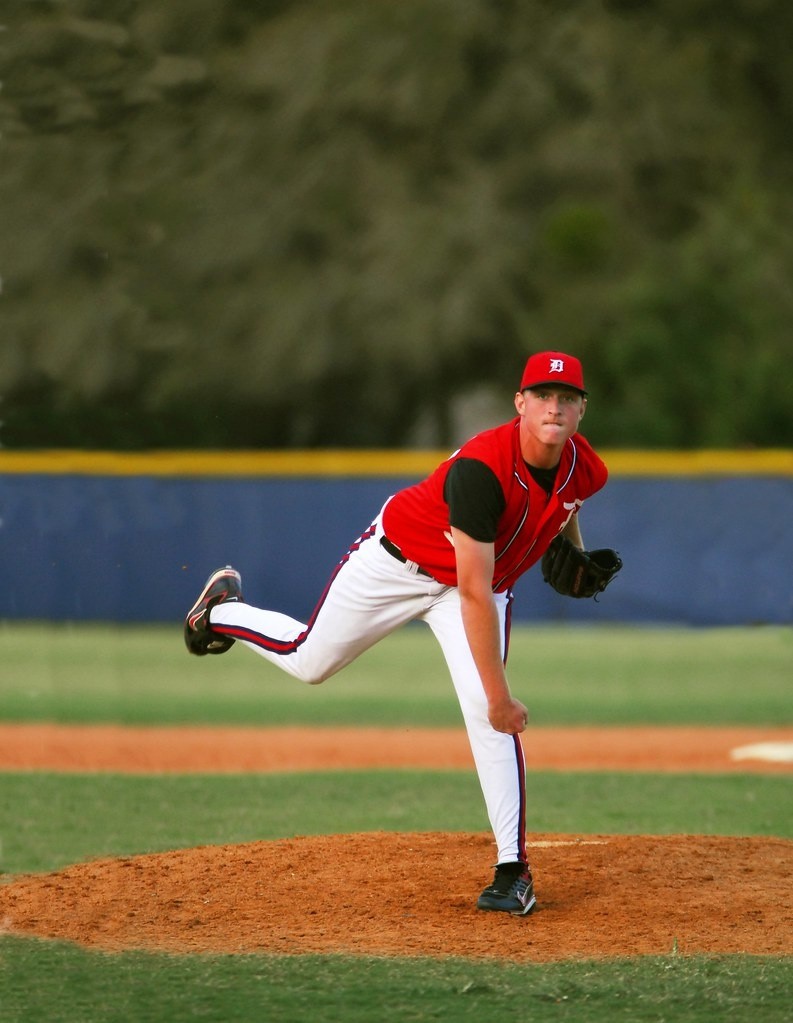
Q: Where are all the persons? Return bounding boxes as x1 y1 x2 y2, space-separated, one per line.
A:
184 351 623 915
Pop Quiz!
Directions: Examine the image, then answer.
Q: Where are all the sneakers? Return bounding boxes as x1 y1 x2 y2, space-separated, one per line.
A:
184 564 244 656
476 860 536 917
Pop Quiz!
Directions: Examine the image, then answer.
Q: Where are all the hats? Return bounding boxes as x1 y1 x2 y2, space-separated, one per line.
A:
519 351 588 399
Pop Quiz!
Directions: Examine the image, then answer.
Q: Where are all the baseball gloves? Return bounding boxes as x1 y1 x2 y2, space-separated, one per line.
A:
543 532 623 599
